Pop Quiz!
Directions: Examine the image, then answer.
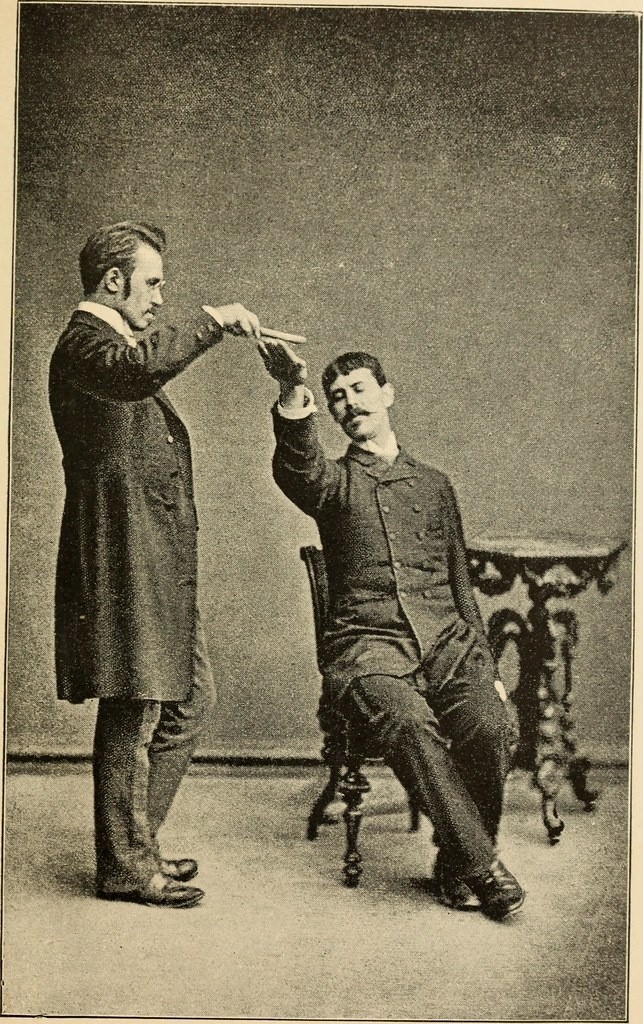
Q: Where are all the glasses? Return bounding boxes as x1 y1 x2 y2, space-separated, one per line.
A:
119 272 167 292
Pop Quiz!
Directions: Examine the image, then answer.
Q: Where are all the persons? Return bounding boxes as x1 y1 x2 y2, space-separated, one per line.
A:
254 334 528 926
47 221 266 913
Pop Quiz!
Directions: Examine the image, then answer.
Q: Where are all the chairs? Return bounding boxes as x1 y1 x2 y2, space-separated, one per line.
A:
299 545 420 891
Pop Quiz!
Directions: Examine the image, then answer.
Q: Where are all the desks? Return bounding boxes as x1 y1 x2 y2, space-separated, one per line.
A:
460 533 626 845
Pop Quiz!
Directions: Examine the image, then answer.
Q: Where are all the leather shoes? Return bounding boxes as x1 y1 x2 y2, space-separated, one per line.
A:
157 857 200 883
432 856 527 922
97 871 206 909
